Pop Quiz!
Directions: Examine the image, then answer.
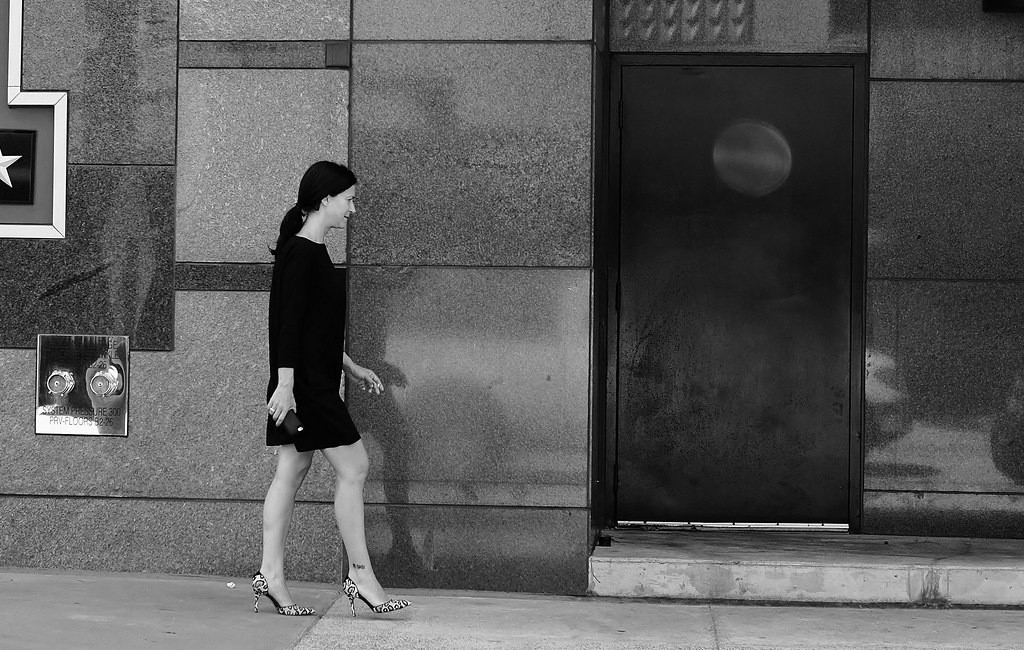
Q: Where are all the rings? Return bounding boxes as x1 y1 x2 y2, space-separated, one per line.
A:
270 408 275 412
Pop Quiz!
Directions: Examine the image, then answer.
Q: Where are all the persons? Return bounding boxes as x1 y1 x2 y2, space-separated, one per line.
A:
251 161 411 618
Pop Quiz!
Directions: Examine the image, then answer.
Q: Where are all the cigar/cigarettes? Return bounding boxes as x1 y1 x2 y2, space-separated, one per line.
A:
378 383 380 388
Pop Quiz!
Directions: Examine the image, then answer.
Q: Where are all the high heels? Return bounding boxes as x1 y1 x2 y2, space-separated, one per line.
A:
343 573 413 617
251 570 316 616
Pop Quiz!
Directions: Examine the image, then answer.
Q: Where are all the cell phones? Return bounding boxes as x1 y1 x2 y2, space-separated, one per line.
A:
283 409 305 436
